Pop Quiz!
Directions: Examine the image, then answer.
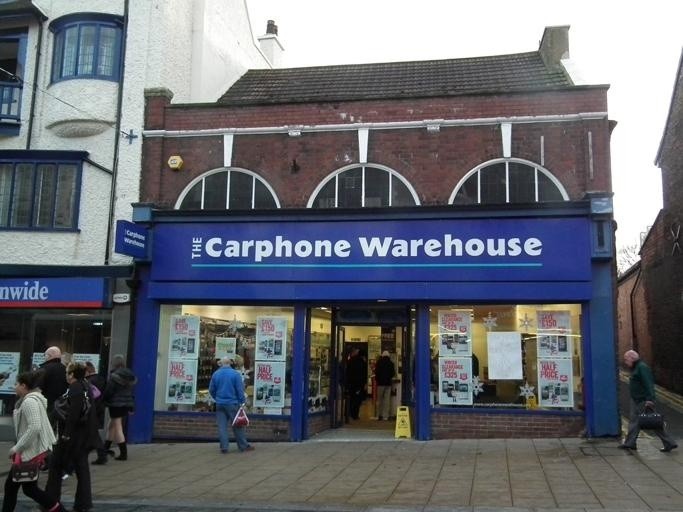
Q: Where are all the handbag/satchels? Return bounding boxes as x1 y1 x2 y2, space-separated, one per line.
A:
54 389 91 421
638 408 663 430
11 462 40 483
88 383 101 399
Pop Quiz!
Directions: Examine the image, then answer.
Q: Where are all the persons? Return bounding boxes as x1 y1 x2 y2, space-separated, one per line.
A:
256 387 263 400
542 385 548 400
187 339 194 353
0 344 138 512
412 345 479 404
347 347 366 420
338 350 353 417
209 356 256 452
558 335 566 351
374 350 394 421
274 340 281 354
615 349 677 452
169 385 175 396
268 385 273 396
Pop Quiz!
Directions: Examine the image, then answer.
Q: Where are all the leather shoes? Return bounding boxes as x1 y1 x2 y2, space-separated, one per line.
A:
660 444 677 451
618 444 637 450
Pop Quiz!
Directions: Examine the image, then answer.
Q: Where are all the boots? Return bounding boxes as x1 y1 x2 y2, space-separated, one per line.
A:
92 441 127 464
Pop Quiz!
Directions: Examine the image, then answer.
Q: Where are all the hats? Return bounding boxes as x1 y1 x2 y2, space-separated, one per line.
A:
382 351 390 357
